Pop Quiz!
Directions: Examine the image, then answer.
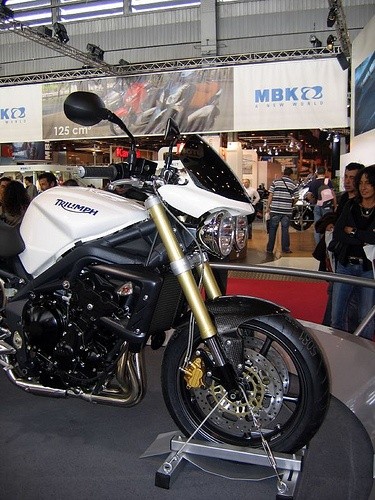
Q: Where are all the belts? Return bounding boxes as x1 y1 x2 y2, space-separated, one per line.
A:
348 256 364 266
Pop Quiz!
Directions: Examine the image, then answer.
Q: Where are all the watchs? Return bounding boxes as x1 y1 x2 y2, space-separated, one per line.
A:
349 228 357 237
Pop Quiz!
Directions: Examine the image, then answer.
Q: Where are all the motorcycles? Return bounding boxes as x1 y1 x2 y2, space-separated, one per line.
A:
289 187 314 231
145 80 223 132
1 92 331 454
110 81 163 136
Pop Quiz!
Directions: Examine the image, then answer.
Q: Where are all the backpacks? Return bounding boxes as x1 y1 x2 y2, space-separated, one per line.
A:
317 177 334 210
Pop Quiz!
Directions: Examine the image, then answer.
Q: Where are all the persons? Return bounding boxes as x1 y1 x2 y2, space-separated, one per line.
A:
325 162 366 274
306 167 338 245
265 167 300 253
242 179 260 239
0 177 11 206
37 173 58 191
23 176 38 202
330 164 375 340
0 181 32 228
57 177 63 186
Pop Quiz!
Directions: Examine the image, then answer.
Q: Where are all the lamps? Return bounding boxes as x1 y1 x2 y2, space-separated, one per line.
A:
327 35 338 50
310 36 321 52
52 22 70 43
337 53 349 70
38 25 52 39
119 58 130 70
87 43 105 59
327 5 337 27
0 6 14 17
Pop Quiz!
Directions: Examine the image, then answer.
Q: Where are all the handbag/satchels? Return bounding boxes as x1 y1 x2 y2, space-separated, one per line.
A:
265 212 279 234
